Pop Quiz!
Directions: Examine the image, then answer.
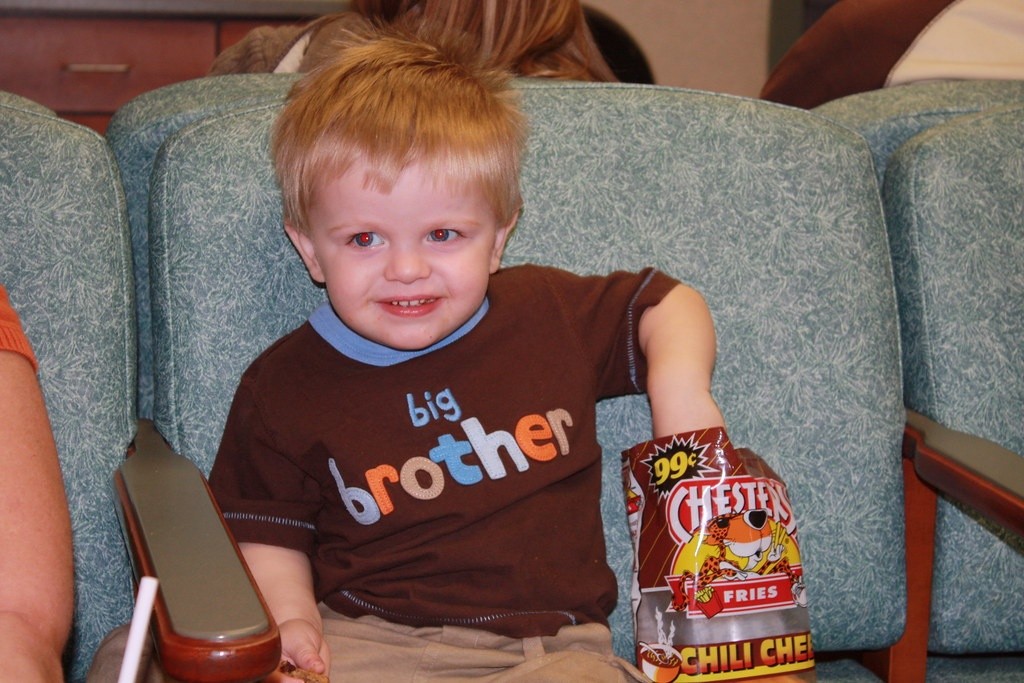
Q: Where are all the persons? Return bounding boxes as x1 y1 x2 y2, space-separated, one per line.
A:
208 0 620 83
758 0 1024 111
208 37 724 683
0 283 74 683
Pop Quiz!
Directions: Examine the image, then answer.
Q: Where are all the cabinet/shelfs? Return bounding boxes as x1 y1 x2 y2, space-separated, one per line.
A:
0 14 315 137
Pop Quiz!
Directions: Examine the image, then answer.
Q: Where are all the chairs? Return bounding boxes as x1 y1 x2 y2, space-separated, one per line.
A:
0 77 1024 683
